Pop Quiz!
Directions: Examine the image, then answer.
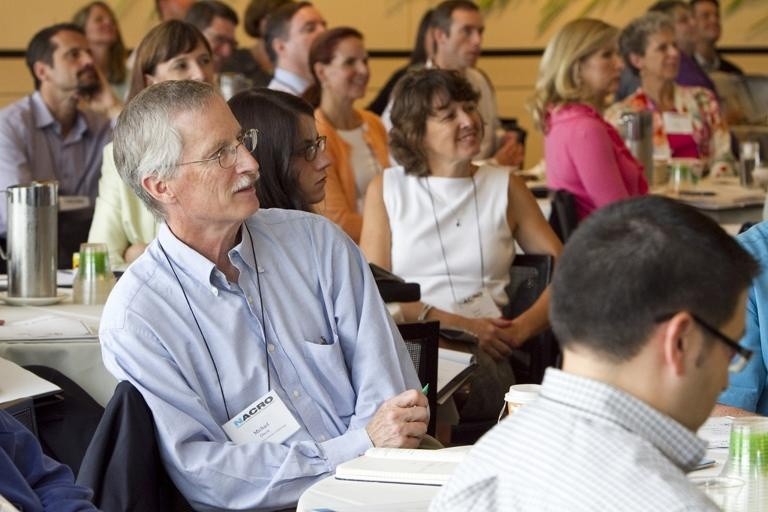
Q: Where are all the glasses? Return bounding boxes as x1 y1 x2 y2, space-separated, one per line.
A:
290 132 328 162
649 308 754 375
161 127 260 171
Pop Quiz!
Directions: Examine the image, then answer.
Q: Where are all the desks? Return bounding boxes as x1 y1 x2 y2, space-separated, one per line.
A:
646 169 768 225
727 123 768 157
296 418 768 512
0 271 117 409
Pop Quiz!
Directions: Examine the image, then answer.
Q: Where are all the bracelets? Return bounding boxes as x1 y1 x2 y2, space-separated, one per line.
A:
415 304 433 321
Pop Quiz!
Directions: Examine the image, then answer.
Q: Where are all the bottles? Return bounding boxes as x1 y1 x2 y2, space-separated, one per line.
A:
617 108 653 187
0 181 58 296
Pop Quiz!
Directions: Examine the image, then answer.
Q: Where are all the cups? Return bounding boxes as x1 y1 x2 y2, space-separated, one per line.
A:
71 242 114 305
668 159 694 195
688 415 767 512
503 383 542 417
738 141 759 188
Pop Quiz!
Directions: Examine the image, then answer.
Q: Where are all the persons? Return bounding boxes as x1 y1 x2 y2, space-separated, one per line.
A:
0 1 391 280
423 192 761 512
97 80 449 511
0 407 102 511
358 67 564 395
523 1 767 228
363 0 495 162
708 219 767 419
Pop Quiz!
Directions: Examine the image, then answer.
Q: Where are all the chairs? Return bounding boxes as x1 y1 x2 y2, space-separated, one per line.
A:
114 380 193 511
1 397 37 436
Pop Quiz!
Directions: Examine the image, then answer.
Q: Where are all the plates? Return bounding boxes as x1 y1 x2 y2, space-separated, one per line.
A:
0 292 69 307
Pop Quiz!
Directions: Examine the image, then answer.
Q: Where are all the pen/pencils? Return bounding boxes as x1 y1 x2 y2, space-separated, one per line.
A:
677 192 717 196
423 383 429 393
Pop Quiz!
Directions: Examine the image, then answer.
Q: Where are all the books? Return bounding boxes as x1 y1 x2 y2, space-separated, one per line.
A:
334 446 462 486
